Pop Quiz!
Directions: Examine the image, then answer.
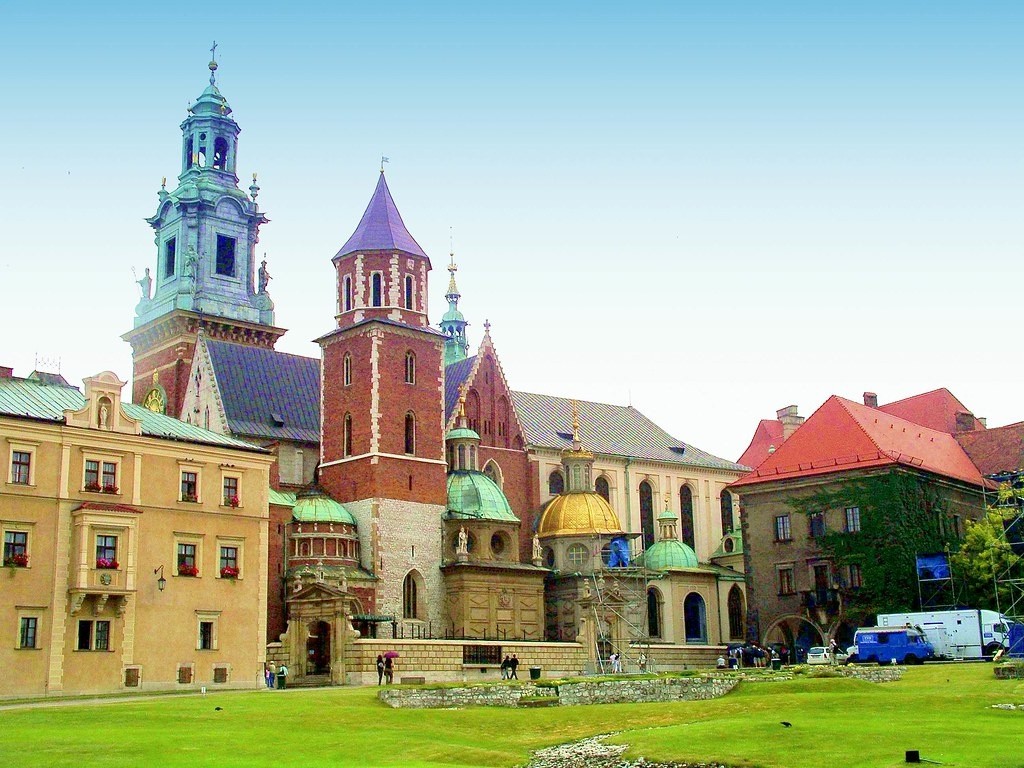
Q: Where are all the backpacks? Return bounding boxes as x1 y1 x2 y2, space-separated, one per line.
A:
501 660 507 669
832 643 838 654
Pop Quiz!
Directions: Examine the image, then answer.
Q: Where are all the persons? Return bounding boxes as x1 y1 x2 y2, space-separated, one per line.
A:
136 267 152 300
828 639 839 666
277 663 288 689
99 402 108 426
615 650 623 673
637 651 644 673
716 654 725 666
458 525 468 554
258 260 270 293
532 534 540 558
844 651 856 665
375 655 384 686
268 660 276 688
384 656 393 685
638 651 647 672
609 651 618 674
726 639 787 673
509 654 519 680
183 243 199 281
265 666 271 687
500 656 510 680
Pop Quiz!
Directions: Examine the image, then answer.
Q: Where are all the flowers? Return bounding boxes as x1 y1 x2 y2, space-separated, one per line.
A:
220 565 240 586
224 494 240 510
84 481 119 495
178 563 199 578
183 492 199 503
96 557 119 570
5 552 29 567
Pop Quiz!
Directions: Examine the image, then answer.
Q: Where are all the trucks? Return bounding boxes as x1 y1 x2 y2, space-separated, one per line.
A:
845 608 1014 662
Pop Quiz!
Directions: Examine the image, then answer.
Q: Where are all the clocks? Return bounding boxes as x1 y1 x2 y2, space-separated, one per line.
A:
142 384 168 417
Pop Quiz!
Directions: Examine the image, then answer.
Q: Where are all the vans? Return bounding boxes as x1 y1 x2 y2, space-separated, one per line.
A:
853 626 933 665
808 646 848 664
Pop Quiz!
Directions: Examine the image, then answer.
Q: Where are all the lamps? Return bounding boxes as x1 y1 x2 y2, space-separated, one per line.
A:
154 565 166 593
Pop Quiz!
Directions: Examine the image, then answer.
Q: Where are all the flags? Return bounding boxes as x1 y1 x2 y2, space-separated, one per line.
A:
382 156 390 162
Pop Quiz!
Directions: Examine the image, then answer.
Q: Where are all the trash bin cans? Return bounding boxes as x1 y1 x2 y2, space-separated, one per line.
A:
528 667 541 680
277 673 287 689
771 658 781 670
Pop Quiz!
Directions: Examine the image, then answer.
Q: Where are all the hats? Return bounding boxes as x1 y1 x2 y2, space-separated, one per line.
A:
270 661 274 663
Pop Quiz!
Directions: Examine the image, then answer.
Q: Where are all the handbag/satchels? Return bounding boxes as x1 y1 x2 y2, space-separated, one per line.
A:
279 671 284 674
378 663 383 668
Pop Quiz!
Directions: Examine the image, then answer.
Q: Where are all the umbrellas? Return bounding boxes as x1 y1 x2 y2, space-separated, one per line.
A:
383 650 399 658
